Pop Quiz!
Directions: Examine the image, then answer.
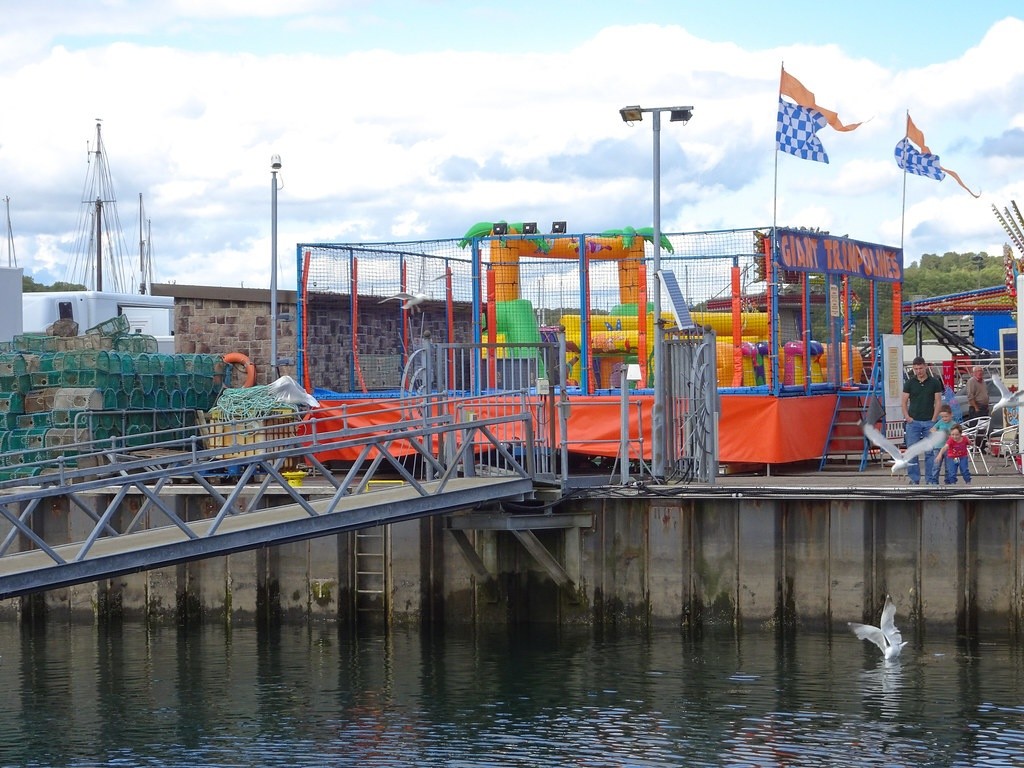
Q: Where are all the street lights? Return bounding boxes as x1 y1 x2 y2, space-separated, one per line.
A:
618 105 695 486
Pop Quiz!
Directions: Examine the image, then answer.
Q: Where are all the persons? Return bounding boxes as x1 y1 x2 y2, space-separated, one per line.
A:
934 424 974 484
966 366 989 455
930 405 957 488
901 357 942 487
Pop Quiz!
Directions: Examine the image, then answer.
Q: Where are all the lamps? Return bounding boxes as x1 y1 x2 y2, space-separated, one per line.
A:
493 223 507 235
523 222 537 234
553 222 566 234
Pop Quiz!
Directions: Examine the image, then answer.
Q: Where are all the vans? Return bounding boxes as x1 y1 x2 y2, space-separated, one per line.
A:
22 290 175 355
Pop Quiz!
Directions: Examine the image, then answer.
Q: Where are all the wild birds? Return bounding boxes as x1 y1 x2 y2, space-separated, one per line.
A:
848 594 909 662
860 419 947 474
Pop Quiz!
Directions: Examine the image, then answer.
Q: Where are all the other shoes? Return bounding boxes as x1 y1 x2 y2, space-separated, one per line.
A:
965 481 971 485
981 450 987 454
910 481 919 485
926 482 938 486
945 483 955 486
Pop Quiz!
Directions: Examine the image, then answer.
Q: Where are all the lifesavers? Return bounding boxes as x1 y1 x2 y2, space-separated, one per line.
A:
213 353 255 390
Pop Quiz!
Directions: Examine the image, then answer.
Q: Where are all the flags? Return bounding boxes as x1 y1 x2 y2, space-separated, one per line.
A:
894 137 946 182
776 99 830 165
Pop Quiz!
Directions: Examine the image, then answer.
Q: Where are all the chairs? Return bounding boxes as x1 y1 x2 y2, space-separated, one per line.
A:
961 415 1020 476
